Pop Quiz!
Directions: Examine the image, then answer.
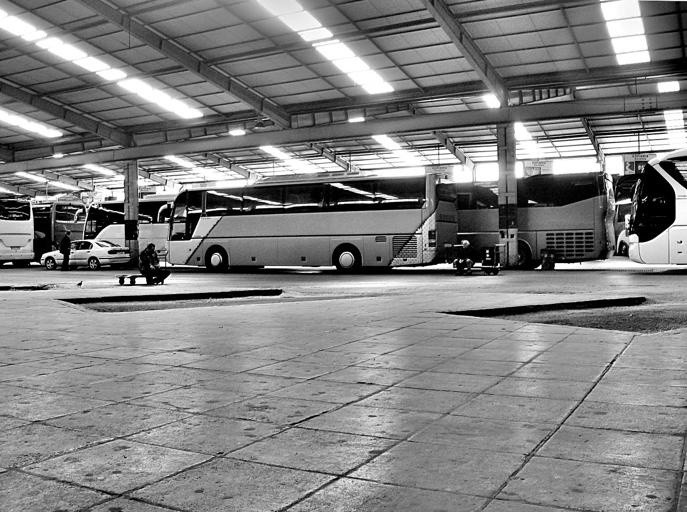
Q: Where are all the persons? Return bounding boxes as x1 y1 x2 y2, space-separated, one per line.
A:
58 229 71 269
452 239 475 272
140 243 171 284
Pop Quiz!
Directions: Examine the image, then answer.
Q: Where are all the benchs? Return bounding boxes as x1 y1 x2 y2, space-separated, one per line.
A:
115 273 164 286
453 263 504 276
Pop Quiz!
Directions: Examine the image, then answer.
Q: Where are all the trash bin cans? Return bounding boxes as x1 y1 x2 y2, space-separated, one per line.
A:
540 248 556 271
